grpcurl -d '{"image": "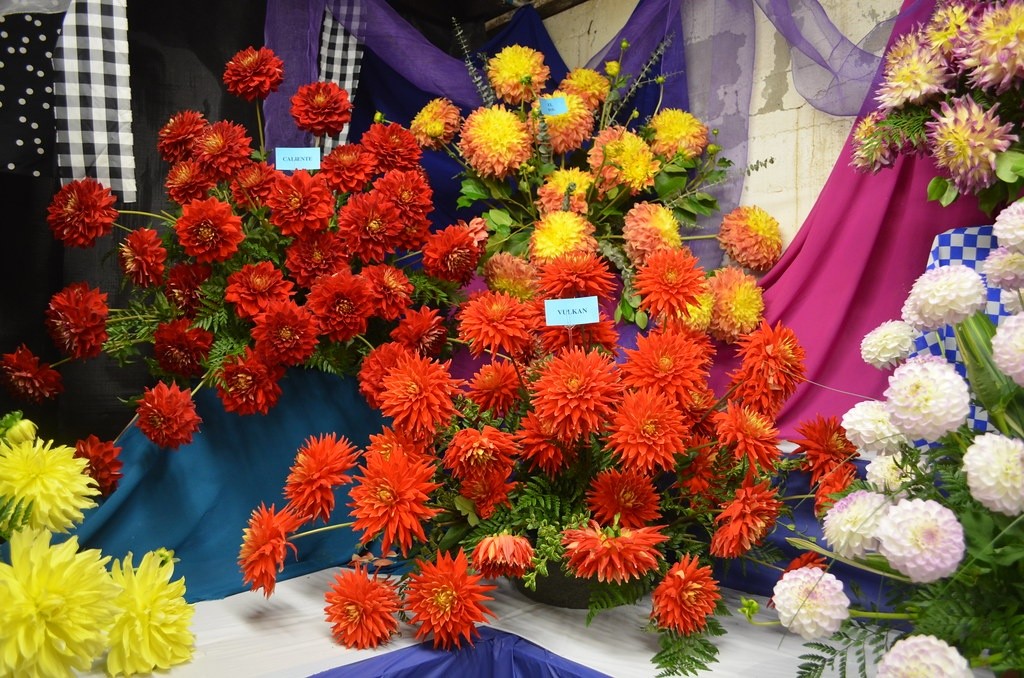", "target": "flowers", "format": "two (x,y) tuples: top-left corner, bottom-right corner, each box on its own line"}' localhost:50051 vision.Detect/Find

(1, 2), (1023, 677)
